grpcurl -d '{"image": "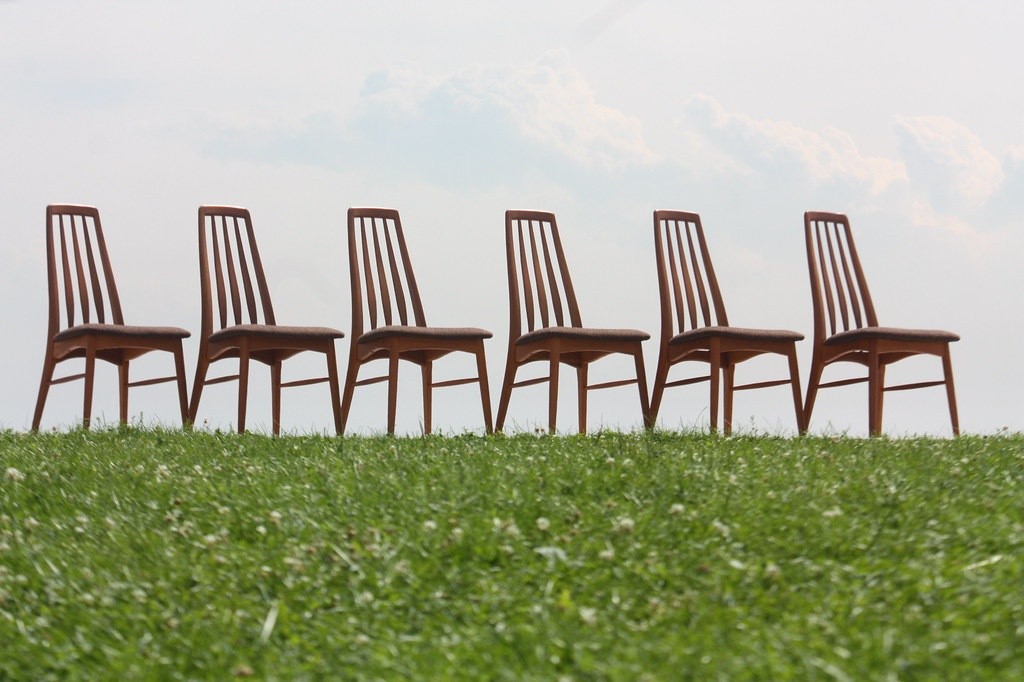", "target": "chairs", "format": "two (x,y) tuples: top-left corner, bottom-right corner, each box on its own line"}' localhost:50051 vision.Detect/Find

(803, 209), (961, 441)
(27, 205), (183, 415)
(496, 210), (651, 437)
(649, 210), (804, 439)
(189, 203), (343, 441)
(340, 207), (495, 439)
